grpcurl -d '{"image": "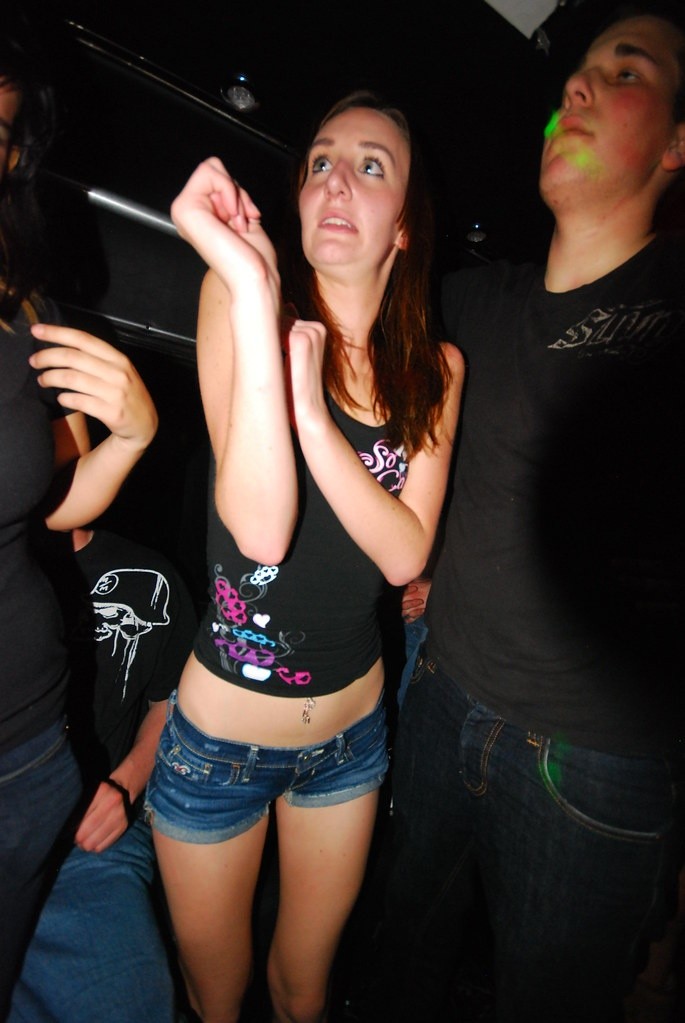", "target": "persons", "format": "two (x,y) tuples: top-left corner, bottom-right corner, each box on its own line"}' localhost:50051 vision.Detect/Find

(0, 12), (685, 1023)
